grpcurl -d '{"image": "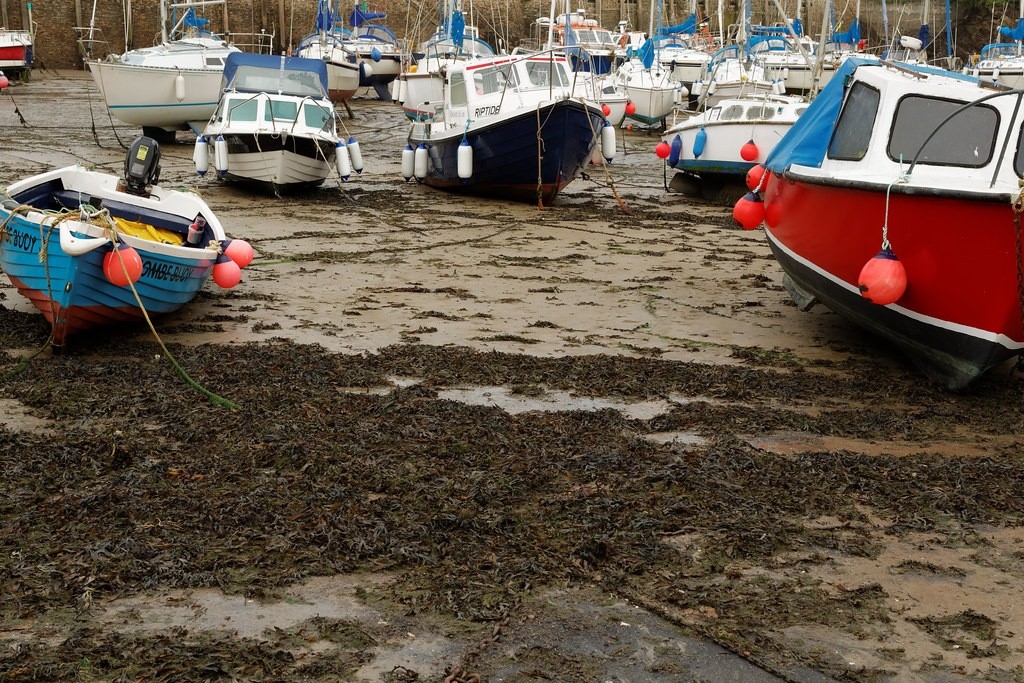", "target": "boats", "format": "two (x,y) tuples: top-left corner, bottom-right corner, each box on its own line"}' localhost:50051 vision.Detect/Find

(293, 0), (1022, 209)
(1, 2), (36, 87)
(76, 0), (246, 143)
(733, 50), (1024, 392)
(0, 136), (253, 356)
(193, 52), (363, 200)
